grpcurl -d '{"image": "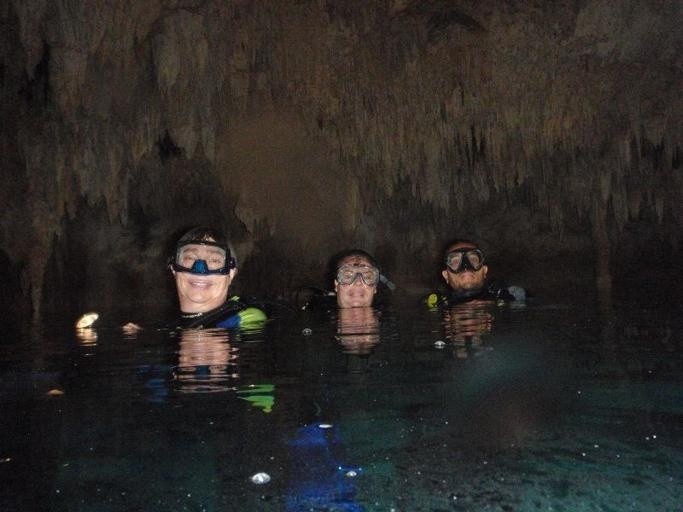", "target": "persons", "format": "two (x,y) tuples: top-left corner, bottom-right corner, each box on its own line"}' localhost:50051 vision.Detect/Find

(328, 246), (383, 311)
(441, 309), (499, 364)
(417, 241), (527, 309)
(333, 310), (386, 363)
(163, 327), (241, 407)
(113, 223), (271, 334)
